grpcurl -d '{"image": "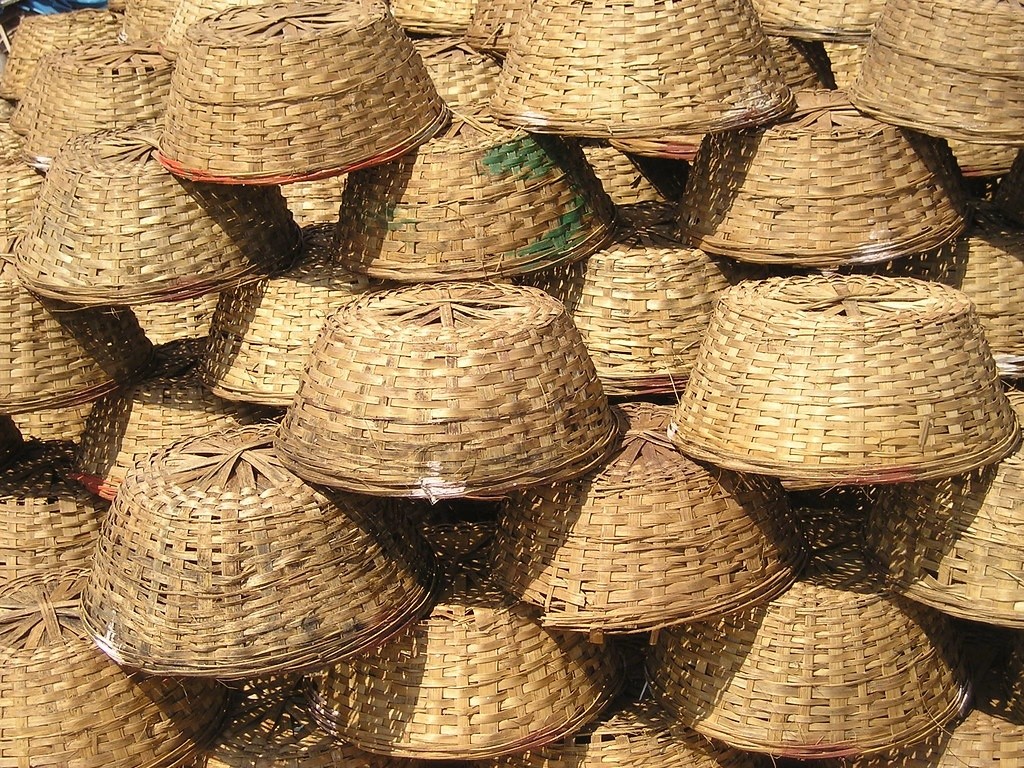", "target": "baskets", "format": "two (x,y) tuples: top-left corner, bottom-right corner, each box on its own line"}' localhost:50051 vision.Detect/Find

(1, 1), (1024, 768)
